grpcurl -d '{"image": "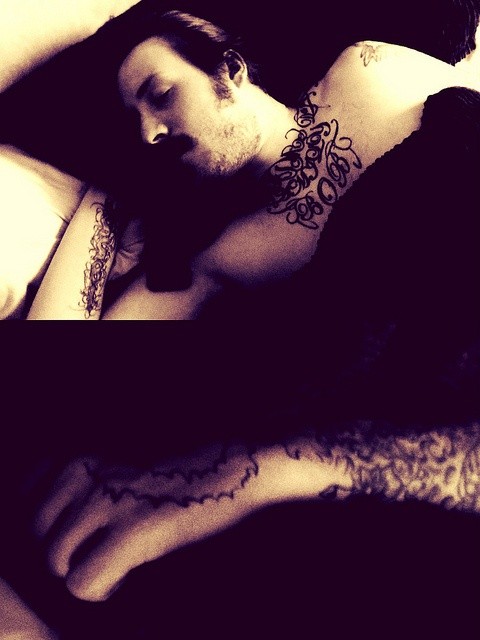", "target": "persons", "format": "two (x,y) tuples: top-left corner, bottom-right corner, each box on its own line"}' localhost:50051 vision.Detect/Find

(23, 7), (480, 604)
